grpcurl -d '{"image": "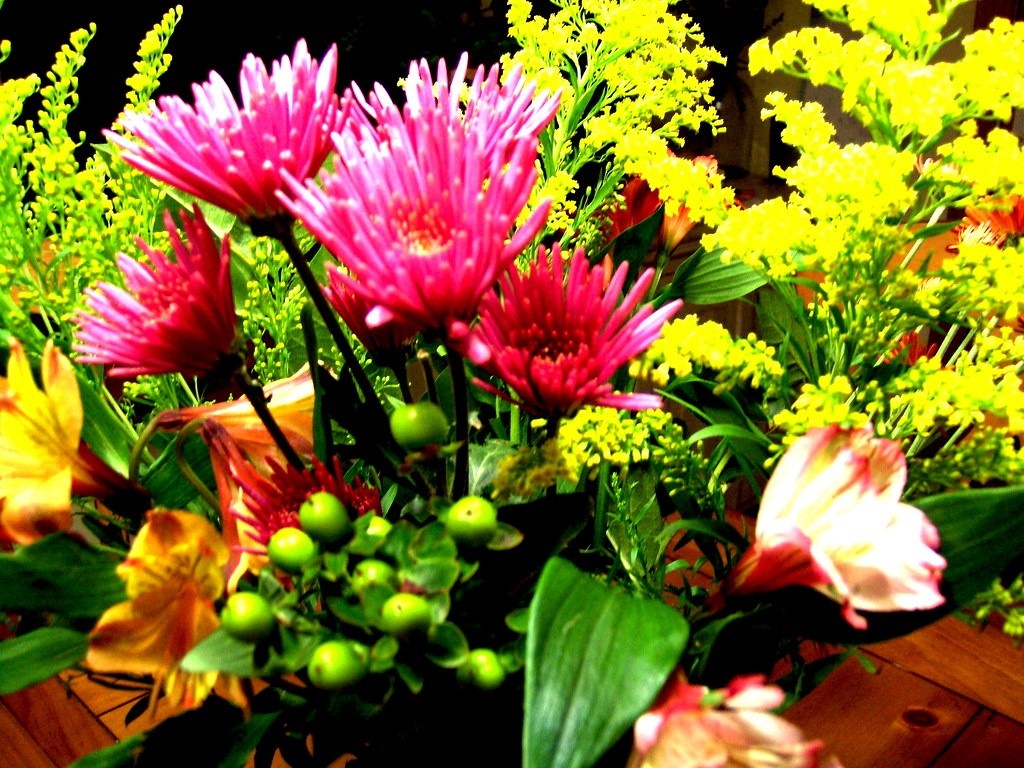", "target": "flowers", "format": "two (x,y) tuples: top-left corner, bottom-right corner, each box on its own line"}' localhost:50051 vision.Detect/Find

(0, 0), (1024, 768)
